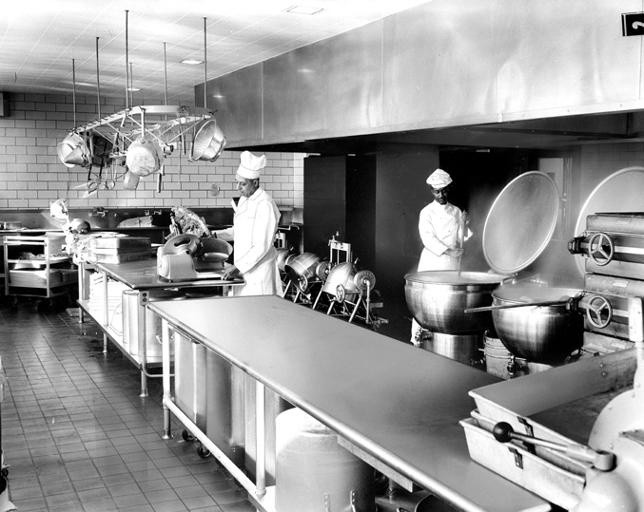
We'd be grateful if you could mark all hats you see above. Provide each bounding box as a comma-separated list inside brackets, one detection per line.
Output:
[425, 168, 454, 191]
[236, 150, 267, 179]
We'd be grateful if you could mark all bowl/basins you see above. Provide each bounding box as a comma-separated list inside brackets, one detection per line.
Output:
[200, 238, 233, 261]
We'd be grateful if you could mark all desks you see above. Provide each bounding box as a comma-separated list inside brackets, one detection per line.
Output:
[140, 293, 554, 511]
[76, 257, 249, 398]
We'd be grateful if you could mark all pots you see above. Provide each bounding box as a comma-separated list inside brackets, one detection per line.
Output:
[126, 108, 164, 177]
[490, 276, 586, 360]
[56, 131, 92, 168]
[403, 269, 512, 332]
[188, 113, 227, 163]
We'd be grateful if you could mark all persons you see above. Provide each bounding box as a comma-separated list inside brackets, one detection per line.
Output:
[408, 180, 474, 348]
[204, 170, 284, 299]
